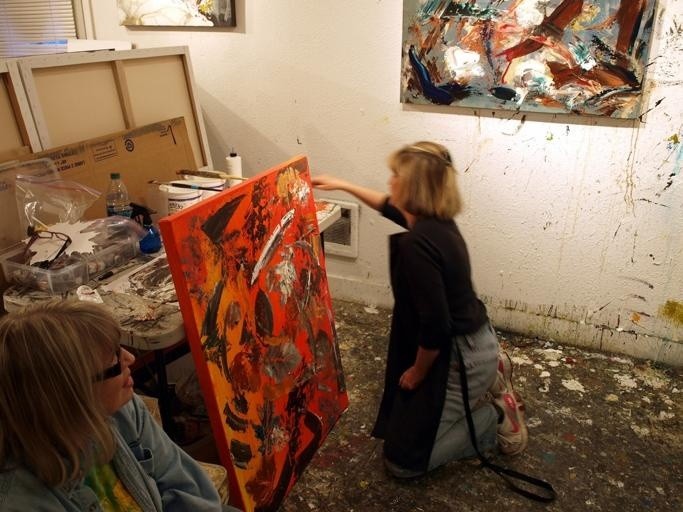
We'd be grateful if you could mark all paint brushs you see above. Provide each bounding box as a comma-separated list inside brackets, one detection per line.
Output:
[175, 169, 249, 180]
[99, 261, 136, 280]
[147, 179, 223, 192]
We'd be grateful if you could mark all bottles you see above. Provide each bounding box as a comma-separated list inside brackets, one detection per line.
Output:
[225, 147, 243, 187]
[105, 173, 132, 218]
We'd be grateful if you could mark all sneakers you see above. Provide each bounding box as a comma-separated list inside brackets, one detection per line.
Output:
[490, 350, 528, 455]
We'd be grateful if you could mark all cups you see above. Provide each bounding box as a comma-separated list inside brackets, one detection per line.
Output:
[142, 186, 169, 226]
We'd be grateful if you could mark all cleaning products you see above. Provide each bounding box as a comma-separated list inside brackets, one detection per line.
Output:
[127, 201, 162, 253]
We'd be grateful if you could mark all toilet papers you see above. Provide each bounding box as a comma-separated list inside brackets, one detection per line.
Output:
[226, 157, 242, 188]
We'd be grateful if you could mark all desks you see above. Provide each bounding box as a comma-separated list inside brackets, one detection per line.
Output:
[3, 203, 344, 444]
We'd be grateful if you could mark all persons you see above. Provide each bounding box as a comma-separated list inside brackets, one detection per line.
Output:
[0, 297, 243, 511]
[310, 140, 528, 480]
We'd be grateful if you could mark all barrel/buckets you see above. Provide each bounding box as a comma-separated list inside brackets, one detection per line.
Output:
[182, 169, 228, 198]
[156, 180, 203, 217]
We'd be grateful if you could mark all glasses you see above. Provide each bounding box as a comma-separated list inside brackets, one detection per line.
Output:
[97, 350, 122, 380]
[24, 231, 72, 265]
